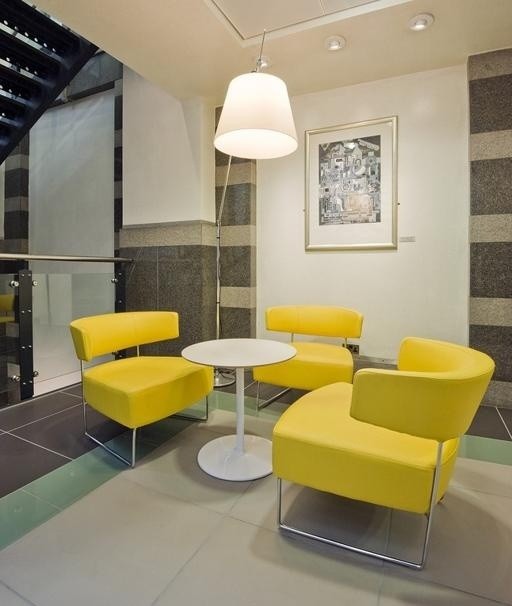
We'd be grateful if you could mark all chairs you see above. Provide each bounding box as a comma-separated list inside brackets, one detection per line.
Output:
[273, 333, 498, 573]
[70, 309, 213, 468]
[252, 304, 364, 415]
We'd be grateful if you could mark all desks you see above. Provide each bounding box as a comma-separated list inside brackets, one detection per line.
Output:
[178, 335, 297, 484]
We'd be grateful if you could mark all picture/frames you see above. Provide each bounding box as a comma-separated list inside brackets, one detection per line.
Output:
[302, 114, 399, 252]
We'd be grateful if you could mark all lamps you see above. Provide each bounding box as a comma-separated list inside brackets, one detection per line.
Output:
[409, 11, 434, 32]
[212, 28, 300, 389]
[322, 36, 347, 53]
[251, 53, 277, 69]
